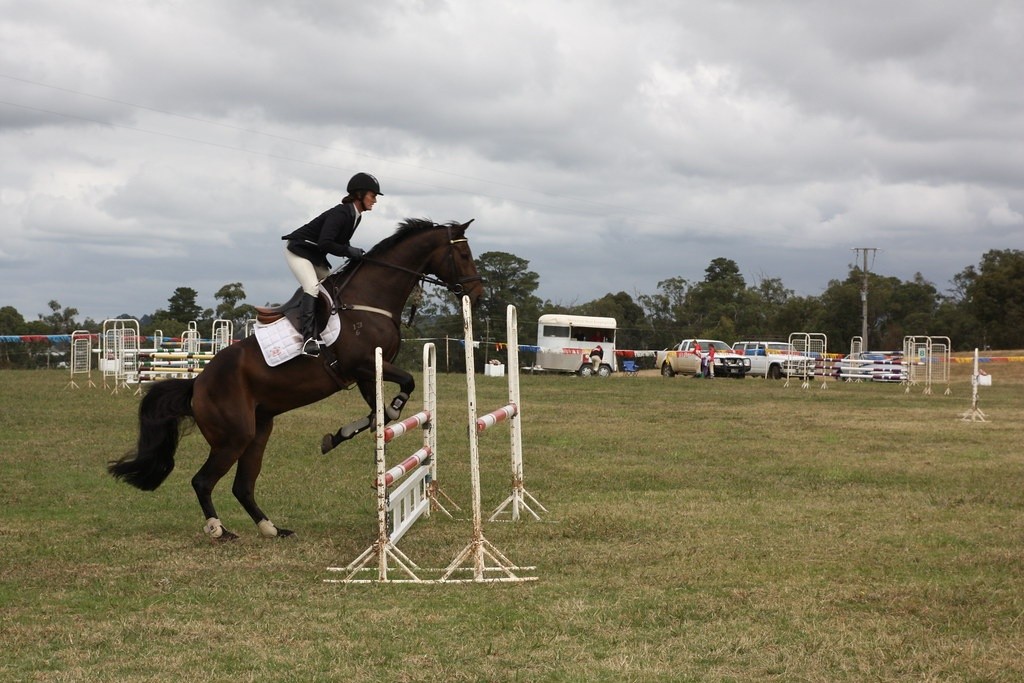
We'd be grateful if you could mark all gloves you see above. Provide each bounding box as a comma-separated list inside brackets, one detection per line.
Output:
[348, 247, 364, 258]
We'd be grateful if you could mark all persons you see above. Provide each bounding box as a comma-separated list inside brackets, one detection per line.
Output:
[282, 173, 384, 353]
[592, 331, 603, 342]
[693, 340, 701, 354]
[590, 346, 603, 375]
[708, 344, 715, 379]
[575, 329, 584, 341]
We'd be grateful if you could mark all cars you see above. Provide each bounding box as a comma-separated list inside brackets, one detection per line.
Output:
[836, 352, 908, 382]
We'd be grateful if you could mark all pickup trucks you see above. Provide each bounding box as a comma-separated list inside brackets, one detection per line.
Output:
[654, 338, 752, 380]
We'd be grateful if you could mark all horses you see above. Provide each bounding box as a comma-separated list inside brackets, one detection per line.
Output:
[108, 218, 487, 539]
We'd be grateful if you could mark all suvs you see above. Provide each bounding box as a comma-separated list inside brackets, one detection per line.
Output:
[730, 341, 814, 382]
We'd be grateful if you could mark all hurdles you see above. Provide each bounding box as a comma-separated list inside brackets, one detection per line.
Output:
[784, 331, 953, 395]
[324, 292, 548, 587]
[66, 317, 234, 397]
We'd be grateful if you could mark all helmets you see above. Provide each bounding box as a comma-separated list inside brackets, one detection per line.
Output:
[347, 173, 383, 195]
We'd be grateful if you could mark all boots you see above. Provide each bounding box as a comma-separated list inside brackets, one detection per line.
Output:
[301, 293, 321, 354]
